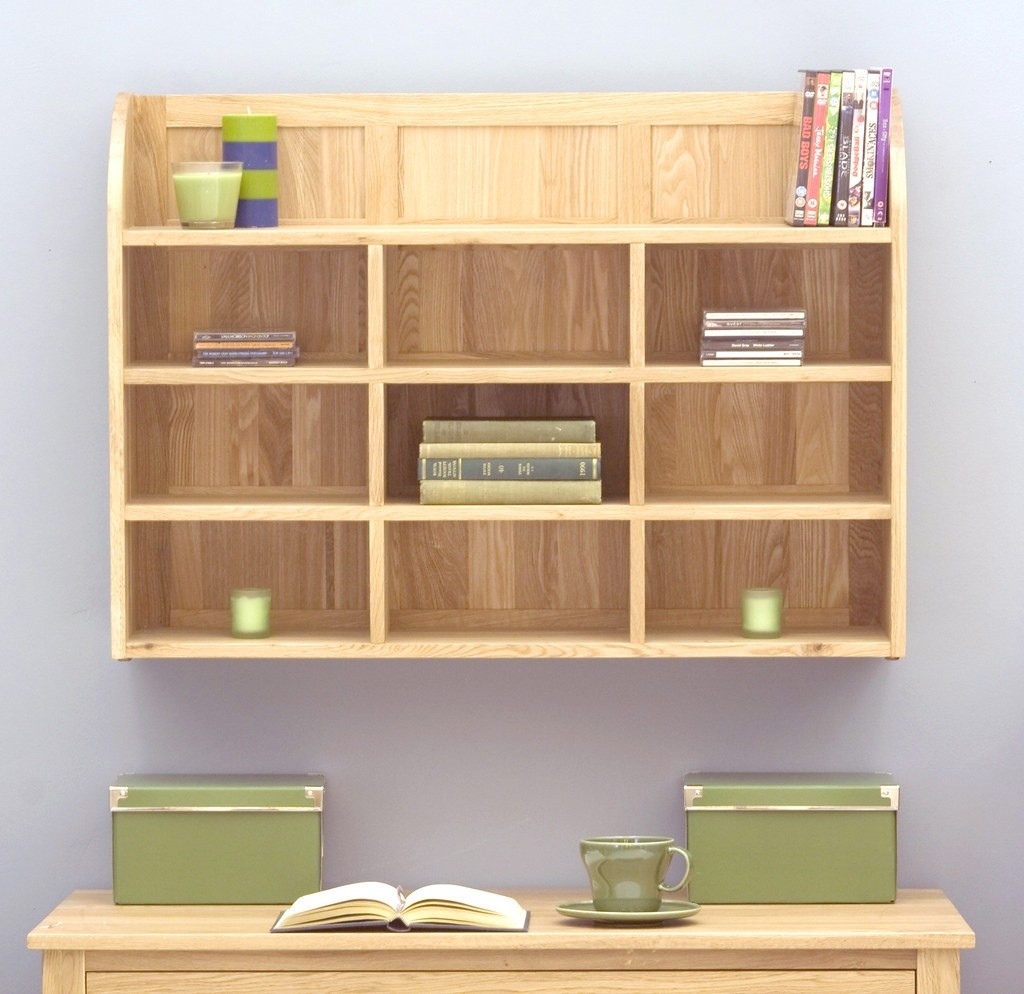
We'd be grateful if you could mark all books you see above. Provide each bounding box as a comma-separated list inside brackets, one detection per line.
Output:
[272, 882, 529, 933]
[418, 417, 603, 505]
[192, 331, 299, 366]
[786, 68, 890, 227]
[701, 309, 805, 365]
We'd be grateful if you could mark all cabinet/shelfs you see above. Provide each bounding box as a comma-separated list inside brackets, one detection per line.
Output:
[26, 888, 975, 994]
[108, 90, 907, 661]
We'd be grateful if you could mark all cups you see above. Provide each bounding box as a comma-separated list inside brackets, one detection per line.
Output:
[580, 835, 690, 912]
[740, 586, 785, 640]
[169, 159, 243, 229]
[229, 587, 270, 640]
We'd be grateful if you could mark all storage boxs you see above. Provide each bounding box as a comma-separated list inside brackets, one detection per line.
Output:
[110, 773, 325, 905]
[682, 771, 899, 904]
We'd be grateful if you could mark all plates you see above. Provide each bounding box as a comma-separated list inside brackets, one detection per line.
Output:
[555, 899, 702, 924]
